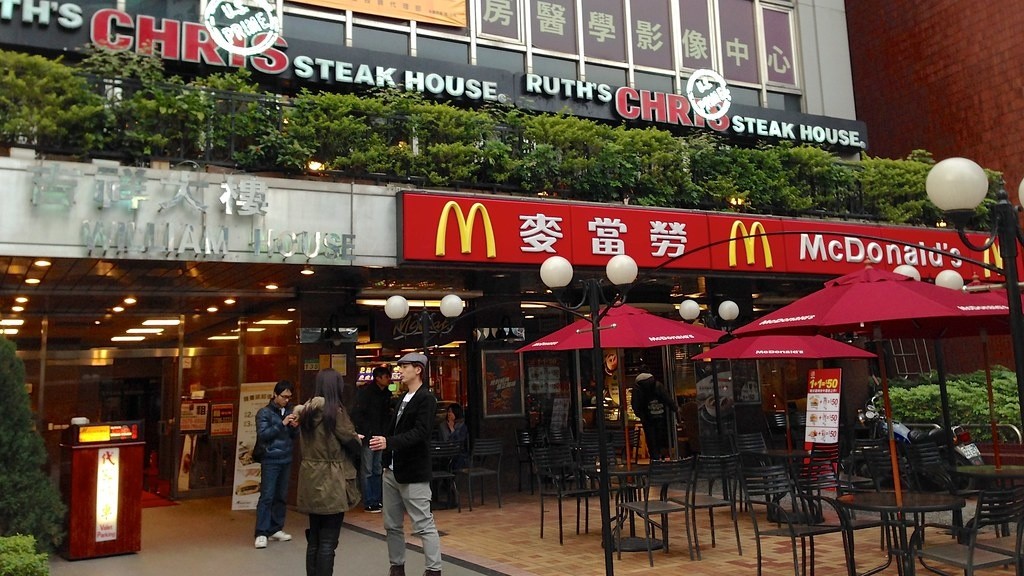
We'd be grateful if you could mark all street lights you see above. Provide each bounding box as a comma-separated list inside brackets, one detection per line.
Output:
[679, 299, 740, 500]
[539, 255, 639, 576]
[925, 157, 1024, 441]
[384, 294, 464, 390]
[893, 263, 964, 538]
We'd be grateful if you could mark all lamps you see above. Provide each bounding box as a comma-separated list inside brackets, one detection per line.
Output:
[496, 315, 524, 343]
[318, 319, 334, 341]
[484, 327, 507, 340]
[322, 312, 353, 346]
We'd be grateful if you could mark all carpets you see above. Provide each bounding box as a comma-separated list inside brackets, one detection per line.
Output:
[142, 490, 180, 507]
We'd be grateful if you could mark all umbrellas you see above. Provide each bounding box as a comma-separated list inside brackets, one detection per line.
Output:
[691, 326, 879, 512]
[733, 255, 1024, 576]
[514, 294, 728, 537]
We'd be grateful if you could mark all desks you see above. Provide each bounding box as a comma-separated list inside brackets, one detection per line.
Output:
[945, 464, 1024, 536]
[580, 464, 664, 552]
[836, 491, 966, 576]
[751, 449, 829, 525]
[548, 440, 599, 498]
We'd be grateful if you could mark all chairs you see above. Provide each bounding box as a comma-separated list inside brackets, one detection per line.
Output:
[427, 409, 1024, 576]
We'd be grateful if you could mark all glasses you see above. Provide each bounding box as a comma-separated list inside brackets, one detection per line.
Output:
[276, 392, 293, 399]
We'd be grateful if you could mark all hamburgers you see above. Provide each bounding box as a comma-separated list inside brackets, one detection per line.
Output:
[810, 414, 817, 422]
[235, 480, 261, 495]
[810, 398, 819, 407]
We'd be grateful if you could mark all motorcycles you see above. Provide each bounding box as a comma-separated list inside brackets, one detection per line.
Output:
[849, 390, 984, 500]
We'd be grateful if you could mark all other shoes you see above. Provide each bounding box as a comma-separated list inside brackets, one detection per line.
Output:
[363, 506, 382, 513]
[389, 563, 409, 576]
[424, 570, 443, 576]
[268, 530, 295, 542]
[254, 535, 269, 548]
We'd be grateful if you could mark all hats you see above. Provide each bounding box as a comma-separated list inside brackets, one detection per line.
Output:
[396, 353, 433, 367]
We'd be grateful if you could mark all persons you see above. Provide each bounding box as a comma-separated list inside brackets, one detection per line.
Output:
[293, 368, 362, 576]
[586, 379, 596, 396]
[350, 367, 391, 513]
[632, 365, 681, 453]
[433, 403, 467, 507]
[357, 353, 441, 576]
[254, 380, 299, 548]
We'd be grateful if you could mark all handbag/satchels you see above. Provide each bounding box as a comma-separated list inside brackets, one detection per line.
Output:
[251, 433, 264, 463]
